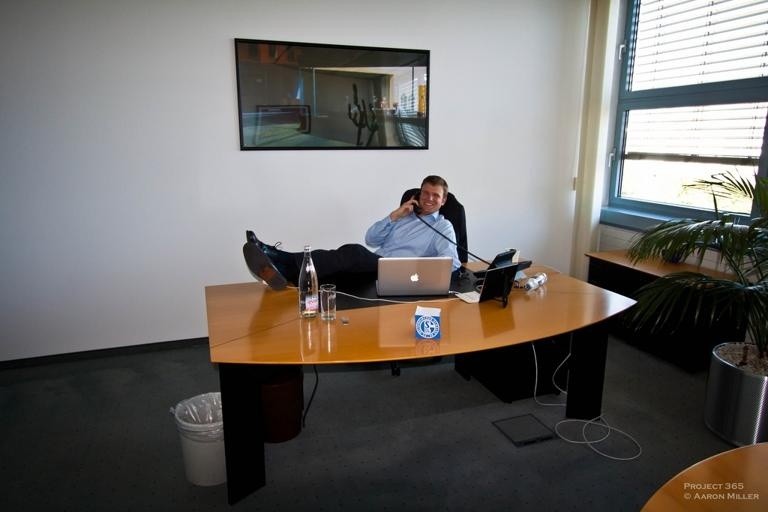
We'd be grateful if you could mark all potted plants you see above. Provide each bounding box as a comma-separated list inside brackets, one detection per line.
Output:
[628, 171, 768, 446]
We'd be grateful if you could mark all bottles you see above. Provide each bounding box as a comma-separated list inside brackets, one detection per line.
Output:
[299, 246, 319, 318]
[523, 272, 548, 289]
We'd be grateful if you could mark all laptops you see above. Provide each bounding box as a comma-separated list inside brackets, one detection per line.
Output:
[377, 301, 451, 349]
[374, 255, 454, 298]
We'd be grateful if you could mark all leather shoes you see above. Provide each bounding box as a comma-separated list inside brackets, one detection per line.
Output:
[243, 230, 287, 291]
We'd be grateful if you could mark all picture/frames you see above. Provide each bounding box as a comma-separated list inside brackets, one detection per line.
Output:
[253, 105, 312, 147]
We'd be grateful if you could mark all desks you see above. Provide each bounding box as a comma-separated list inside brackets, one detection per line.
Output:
[205, 256, 639, 505]
[584, 246, 750, 376]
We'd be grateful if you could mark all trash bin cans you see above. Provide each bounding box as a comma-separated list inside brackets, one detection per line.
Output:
[169, 392, 228, 487]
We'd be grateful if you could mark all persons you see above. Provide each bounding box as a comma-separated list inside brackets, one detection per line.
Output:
[243, 172, 464, 290]
[392, 101, 408, 118]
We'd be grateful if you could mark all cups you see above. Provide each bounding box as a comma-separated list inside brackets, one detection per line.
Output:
[318, 283, 336, 321]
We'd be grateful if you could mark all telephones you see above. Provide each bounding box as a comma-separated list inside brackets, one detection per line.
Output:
[414, 191, 422, 216]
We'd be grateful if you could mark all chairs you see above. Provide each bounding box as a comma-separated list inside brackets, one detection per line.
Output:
[400, 187, 468, 264]
